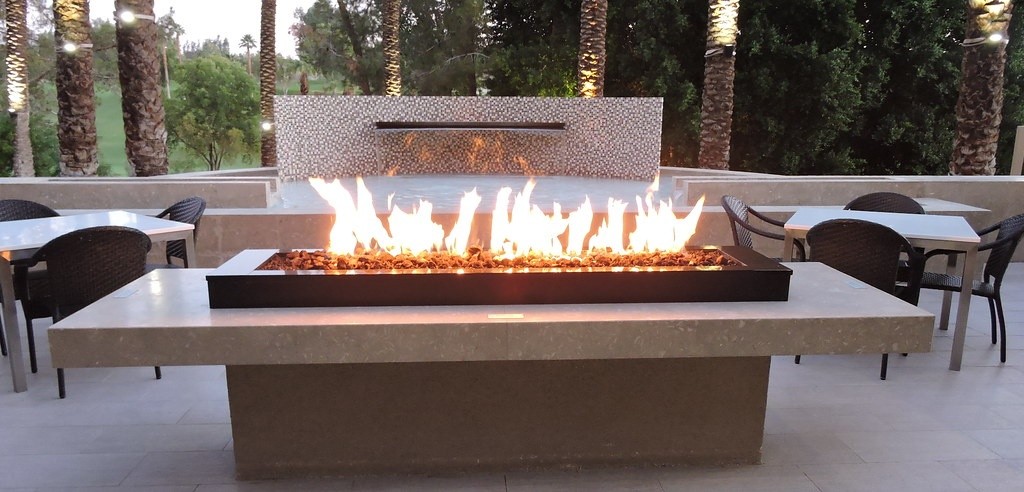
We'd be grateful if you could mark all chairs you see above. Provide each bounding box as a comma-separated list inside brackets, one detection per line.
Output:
[145, 197, 207, 273]
[720, 195, 807, 263]
[794, 219, 928, 381]
[14, 225, 162, 400]
[0, 198, 62, 358]
[843, 191, 924, 280]
[922, 214, 1024, 362]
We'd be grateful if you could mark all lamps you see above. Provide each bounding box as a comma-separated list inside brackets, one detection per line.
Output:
[984, 0, 1005, 12]
[724, 43, 735, 58]
[989, 31, 1003, 41]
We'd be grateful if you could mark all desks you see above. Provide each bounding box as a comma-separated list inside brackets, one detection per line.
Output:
[781, 205, 983, 373]
[0, 211, 195, 393]
